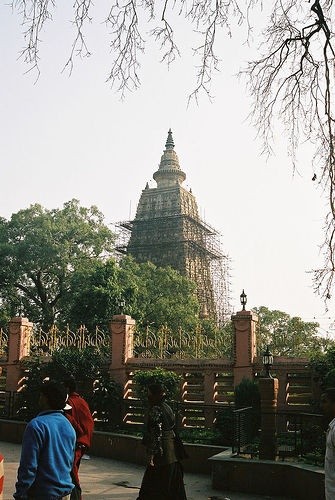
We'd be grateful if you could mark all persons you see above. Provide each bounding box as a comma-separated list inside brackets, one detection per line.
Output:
[12, 381, 78, 500]
[134, 382, 187, 500]
[53, 373, 95, 500]
[323, 418, 335, 499]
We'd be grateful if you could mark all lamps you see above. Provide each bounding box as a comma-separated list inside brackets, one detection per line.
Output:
[239, 288, 248, 311]
[263, 344, 273, 378]
